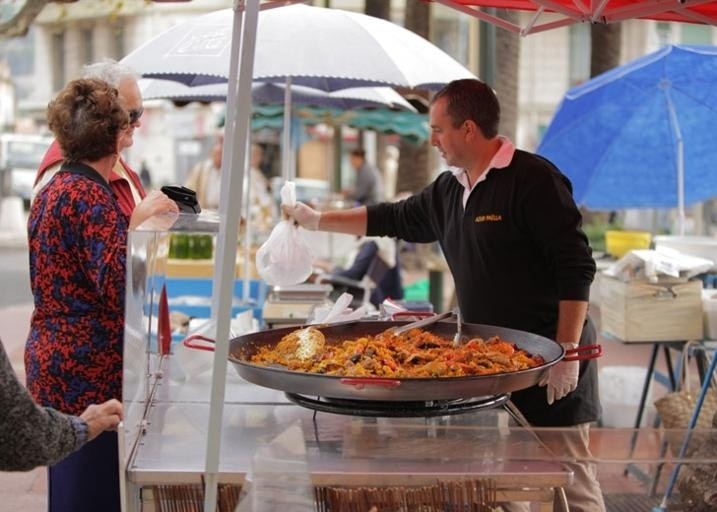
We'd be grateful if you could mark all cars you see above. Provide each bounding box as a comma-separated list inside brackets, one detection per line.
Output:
[4, 128, 151, 213]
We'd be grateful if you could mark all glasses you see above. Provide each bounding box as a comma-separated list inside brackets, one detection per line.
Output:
[130, 108, 143, 119]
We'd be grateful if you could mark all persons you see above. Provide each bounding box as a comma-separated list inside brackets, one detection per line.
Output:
[280, 80, 607, 512]
[0, 338, 124, 472]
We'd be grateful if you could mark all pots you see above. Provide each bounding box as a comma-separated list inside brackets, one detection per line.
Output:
[182, 306, 605, 405]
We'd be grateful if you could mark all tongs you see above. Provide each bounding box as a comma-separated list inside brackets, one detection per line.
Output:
[395, 309, 463, 348]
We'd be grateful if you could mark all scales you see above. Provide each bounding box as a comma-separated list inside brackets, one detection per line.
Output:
[262, 283, 334, 330]
